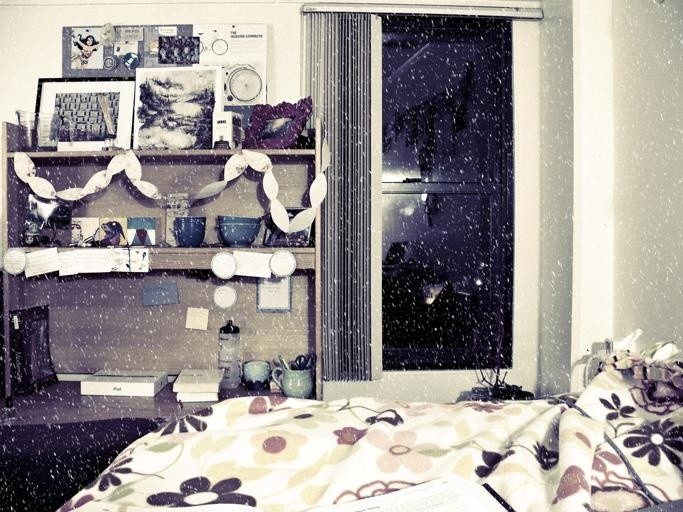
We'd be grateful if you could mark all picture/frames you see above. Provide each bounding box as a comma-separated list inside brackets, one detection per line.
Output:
[33, 77, 136, 150]
[133, 65, 225, 150]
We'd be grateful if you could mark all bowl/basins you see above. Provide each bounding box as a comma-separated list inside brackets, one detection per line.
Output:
[217, 223, 261, 243]
[217, 215, 260, 227]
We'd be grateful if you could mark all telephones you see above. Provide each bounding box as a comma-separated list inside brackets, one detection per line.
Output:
[262, 207, 313, 246]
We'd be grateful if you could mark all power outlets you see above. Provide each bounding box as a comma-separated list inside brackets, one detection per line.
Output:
[591, 342, 607, 358]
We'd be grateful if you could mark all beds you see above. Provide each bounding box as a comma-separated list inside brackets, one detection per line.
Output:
[53, 353, 683, 512]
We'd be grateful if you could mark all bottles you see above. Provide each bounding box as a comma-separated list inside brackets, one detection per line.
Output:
[218, 318, 240, 388]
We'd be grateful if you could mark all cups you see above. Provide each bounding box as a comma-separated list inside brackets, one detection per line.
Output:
[176, 216, 207, 249]
[244, 361, 270, 385]
[272, 363, 313, 399]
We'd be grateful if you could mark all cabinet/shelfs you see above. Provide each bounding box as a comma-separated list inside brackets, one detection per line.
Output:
[0, 118, 321, 511]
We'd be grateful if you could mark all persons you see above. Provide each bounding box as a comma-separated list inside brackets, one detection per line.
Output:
[159, 37, 199, 64]
[71, 35, 103, 69]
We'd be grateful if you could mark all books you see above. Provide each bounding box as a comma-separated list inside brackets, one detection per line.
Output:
[171, 368, 225, 403]
[10, 303, 59, 395]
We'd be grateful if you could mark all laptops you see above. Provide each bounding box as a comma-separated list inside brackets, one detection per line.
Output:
[382, 241, 408, 268]
